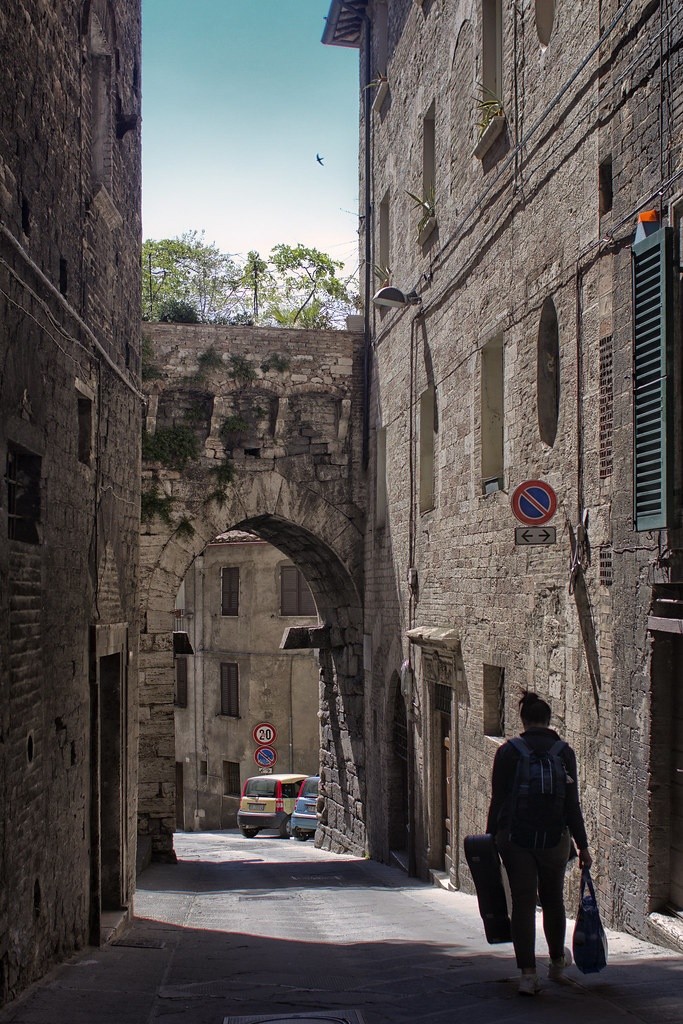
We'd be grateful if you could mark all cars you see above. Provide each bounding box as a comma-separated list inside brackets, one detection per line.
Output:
[290, 776, 320, 841]
[237, 773, 310, 840]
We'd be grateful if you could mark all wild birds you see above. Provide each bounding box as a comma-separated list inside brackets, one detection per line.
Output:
[316, 153, 324, 166]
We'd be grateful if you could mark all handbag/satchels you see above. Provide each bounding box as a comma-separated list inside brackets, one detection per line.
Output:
[573, 865, 607, 974]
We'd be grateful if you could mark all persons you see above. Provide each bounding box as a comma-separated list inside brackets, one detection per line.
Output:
[486, 689, 592, 997]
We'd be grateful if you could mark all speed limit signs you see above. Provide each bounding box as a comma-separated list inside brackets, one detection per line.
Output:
[253, 723, 277, 745]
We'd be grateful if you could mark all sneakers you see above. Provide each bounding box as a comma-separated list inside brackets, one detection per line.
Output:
[519, 973, 545, 993]
[547, 947, 572, 980]
